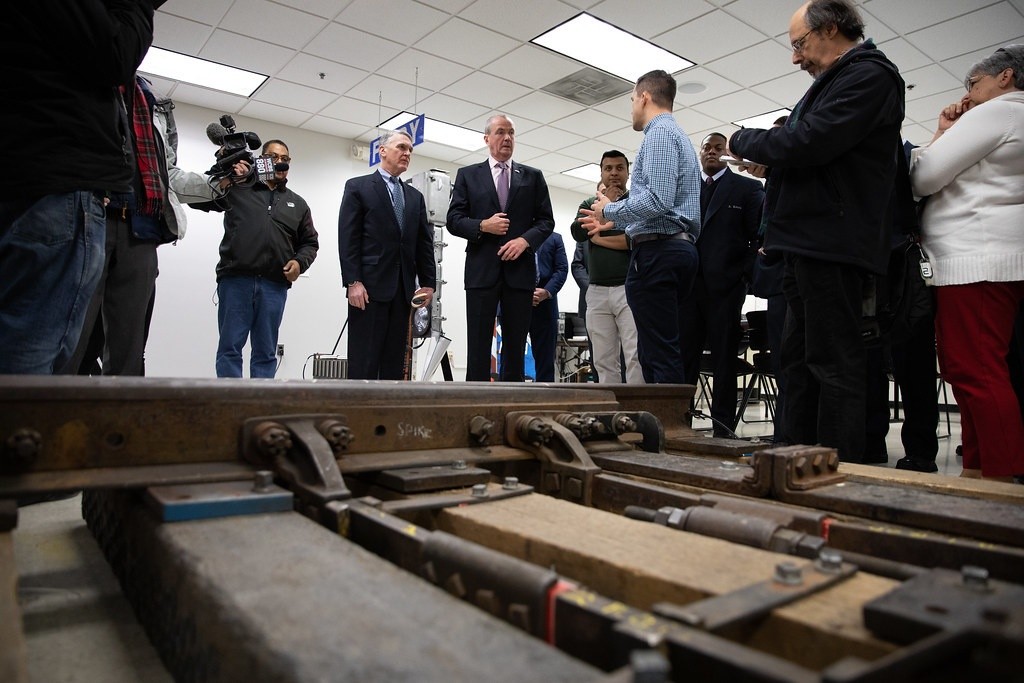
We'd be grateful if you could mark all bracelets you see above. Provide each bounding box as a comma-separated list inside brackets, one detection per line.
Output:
[480, 221, 482, 231]
[602, 208, 605, 219]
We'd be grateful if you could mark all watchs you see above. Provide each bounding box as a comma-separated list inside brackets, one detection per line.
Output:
[349, 281, 358, 287]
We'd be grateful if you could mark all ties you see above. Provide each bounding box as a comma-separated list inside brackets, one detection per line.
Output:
[707, 177, 713, 185]
[389, 176, 404, 233]
[495, 162, 510, 213]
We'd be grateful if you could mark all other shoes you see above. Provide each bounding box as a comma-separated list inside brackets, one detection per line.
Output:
[897, 455, 940, 473]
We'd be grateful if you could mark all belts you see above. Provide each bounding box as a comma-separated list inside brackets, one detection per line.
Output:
[106, 203, 129, 221]
[630, 232, 691, 250]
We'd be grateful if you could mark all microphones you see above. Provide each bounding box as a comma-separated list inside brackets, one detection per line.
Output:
[253, 156, 290, 181]
[206, 123, 228, 146]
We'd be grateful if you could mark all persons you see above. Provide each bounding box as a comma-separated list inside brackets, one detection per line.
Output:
[699, 132, 765, 439]
[590, 69, 700, 383]
[0, 0, 186, 378]
[497, 232, 568, 383]
[887, 131, 939, 473]
[338, 129, 436, 381]
[446, 115, 555, 383]
[571, 181, 626, 383]
[909, 44, 1024, 483]
[570, 148, 645, 383]
[727, 0, 906, 463]
[187, 140, 319, 378]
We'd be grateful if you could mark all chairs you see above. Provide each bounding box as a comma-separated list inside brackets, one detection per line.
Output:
[741, 309, 785, 424]
[694, 319, 751, 418]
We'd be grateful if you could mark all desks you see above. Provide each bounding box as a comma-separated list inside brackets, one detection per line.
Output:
[557, 341, 589, 383]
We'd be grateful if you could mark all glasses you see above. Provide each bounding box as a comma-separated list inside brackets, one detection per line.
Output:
[262, 152, 291, 164]
[967, 72, 992, 91]
[792, 25, 823, 52]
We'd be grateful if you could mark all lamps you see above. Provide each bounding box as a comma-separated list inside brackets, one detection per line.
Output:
[350, 144, 369, 161]
